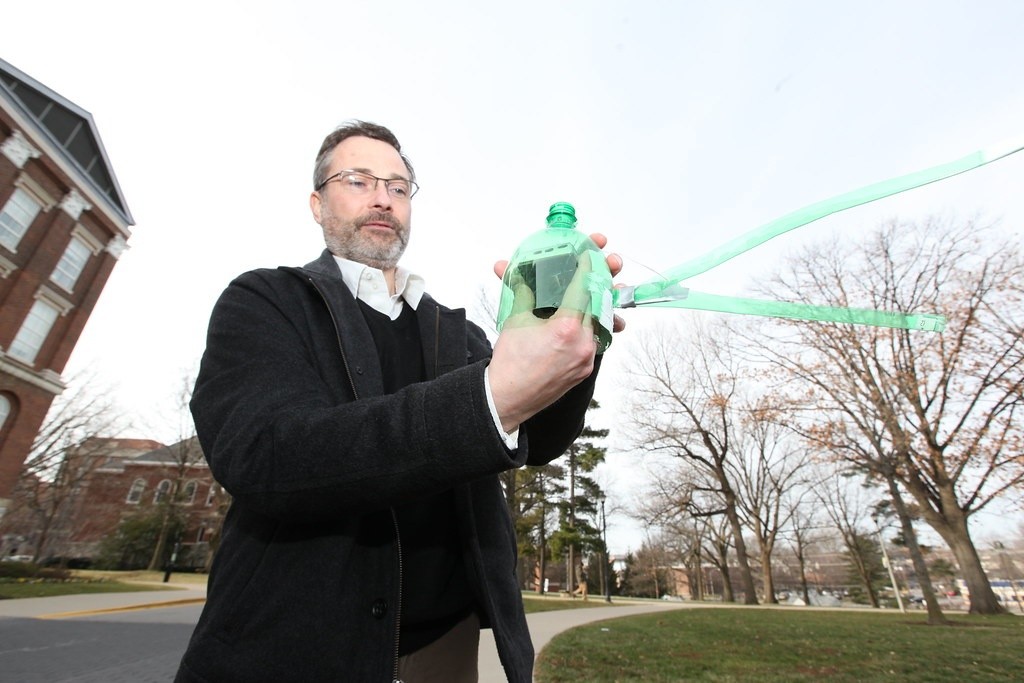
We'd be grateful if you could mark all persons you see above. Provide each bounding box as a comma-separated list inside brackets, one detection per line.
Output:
[572, 562, 588, 601]
[172, 120, 629, 683]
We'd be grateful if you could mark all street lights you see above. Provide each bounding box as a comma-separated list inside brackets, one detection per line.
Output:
[870, 512, 904, 612]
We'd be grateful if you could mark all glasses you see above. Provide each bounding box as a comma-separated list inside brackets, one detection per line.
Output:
[315, 169, 419, 200]
